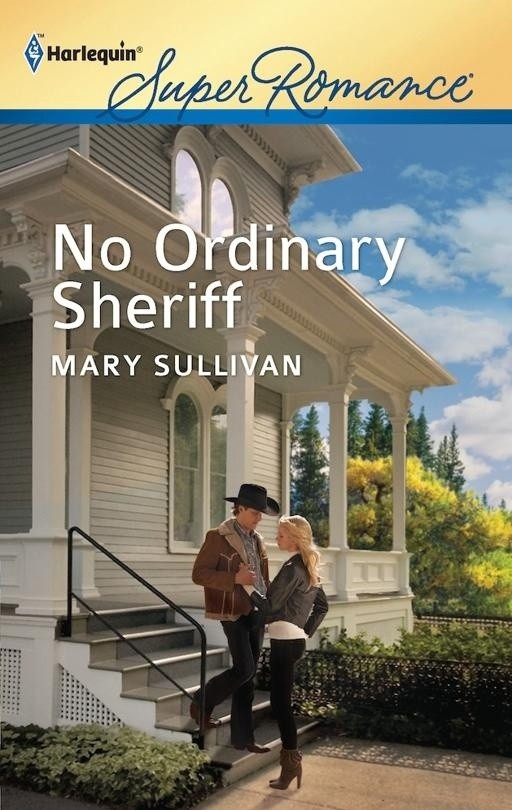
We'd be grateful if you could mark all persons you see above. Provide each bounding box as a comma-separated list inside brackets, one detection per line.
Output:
[181, 483, 280, 753]
[239, 513, 328, 792]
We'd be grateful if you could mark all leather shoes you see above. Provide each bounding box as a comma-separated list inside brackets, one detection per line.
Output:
[234, 742, 271, 753]
[190, 703, 222, 728]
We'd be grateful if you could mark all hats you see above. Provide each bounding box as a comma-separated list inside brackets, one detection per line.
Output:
[224, 484, 280, 516]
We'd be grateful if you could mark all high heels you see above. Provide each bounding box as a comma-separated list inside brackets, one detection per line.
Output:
[269, 749, 302, 789]
